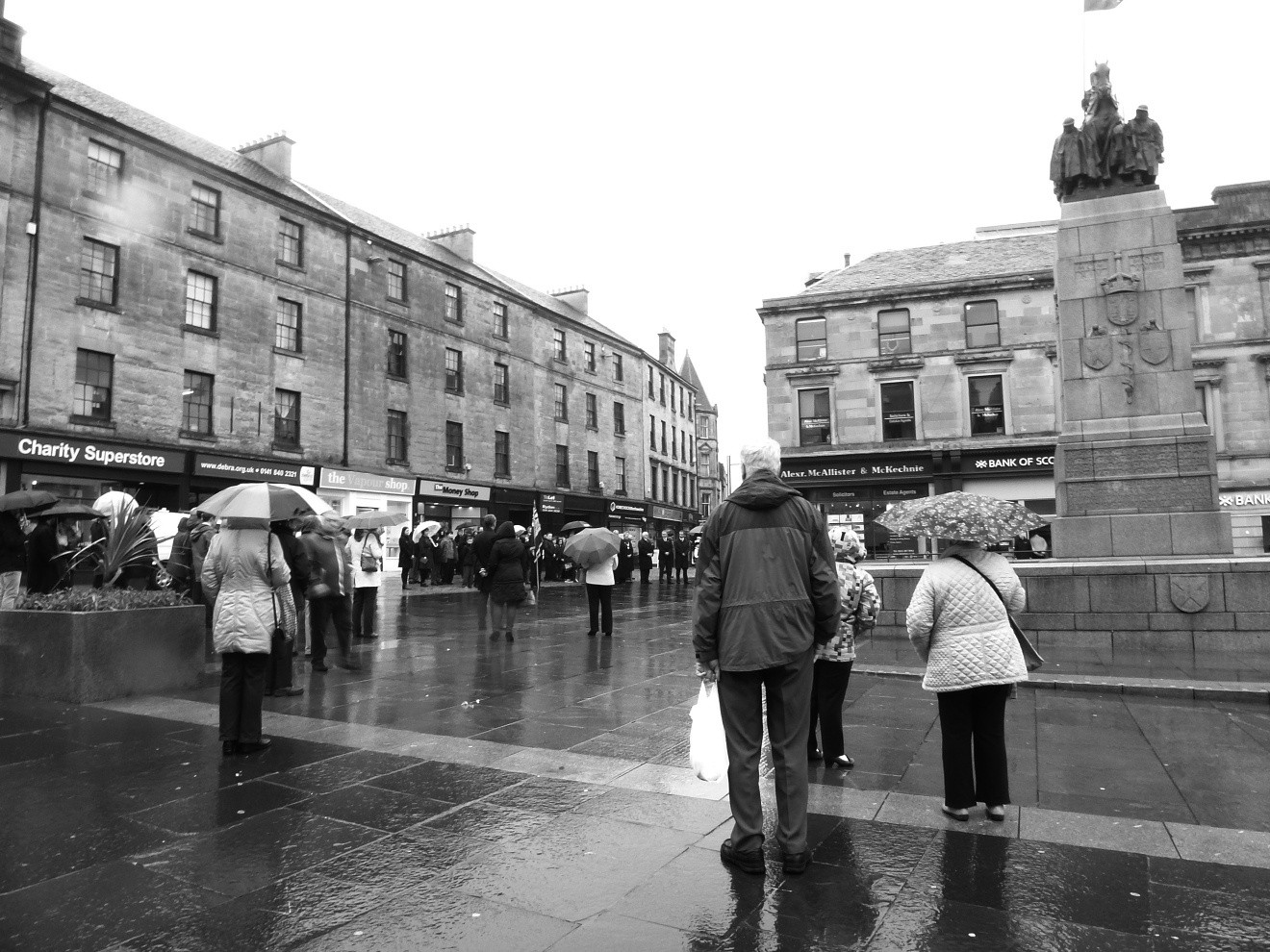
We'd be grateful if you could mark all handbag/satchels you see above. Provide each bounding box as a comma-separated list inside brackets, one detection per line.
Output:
[360, 532, 379, 571]
[517, 589, 535, 609]
[1008, 613, 1044, 672]
[272, 625, 286, 660]
[305, 577, 339, 600]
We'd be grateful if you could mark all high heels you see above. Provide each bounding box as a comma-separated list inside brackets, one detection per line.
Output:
[808, 750, 823, 762]
[587, 629, 596, 637]
[823, 753, 855, 768]
[604, 631, 611, 637]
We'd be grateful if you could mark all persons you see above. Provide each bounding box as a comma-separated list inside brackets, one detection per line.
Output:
[1030, 529, 1048, 558]
[0, 506, 704, 697]
[691, 436, 841, 875]
[1014, 534, 1031, 558]
[199, 517, 292, 756]
[1049, 73, 1165, 195]
[807, 526, 881, 767]
[906, 541, 1030, 821]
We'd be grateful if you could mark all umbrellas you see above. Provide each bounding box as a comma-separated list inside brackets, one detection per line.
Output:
[0, 490, 60, 524]
[194, 481, 334, 520]
[27, 503, 105, 531]
[91, 486, 140, 517]
[872, 489, 1051, 544]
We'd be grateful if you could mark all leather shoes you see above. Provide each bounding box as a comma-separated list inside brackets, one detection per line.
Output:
[720, 838, 766, 873]
[234, 737, 272, 754]
[222, 740, 236, 755]
[782, 849, 810, 873]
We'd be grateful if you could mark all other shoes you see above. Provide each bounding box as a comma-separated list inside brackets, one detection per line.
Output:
[478, 625, 486, 631]
[262, 687, 273, 695]
[985, 804, 1004, 821]
[941, 803, 969, 821]
[292, 629, 380, 656]
[398, 571, 703, 591]
[312, 660, 328, 671]
[339, 655, 359, 668]
[489, 630, 500, 641]
[506, 632, 514, 642]
[274, 686, 303, 697]
